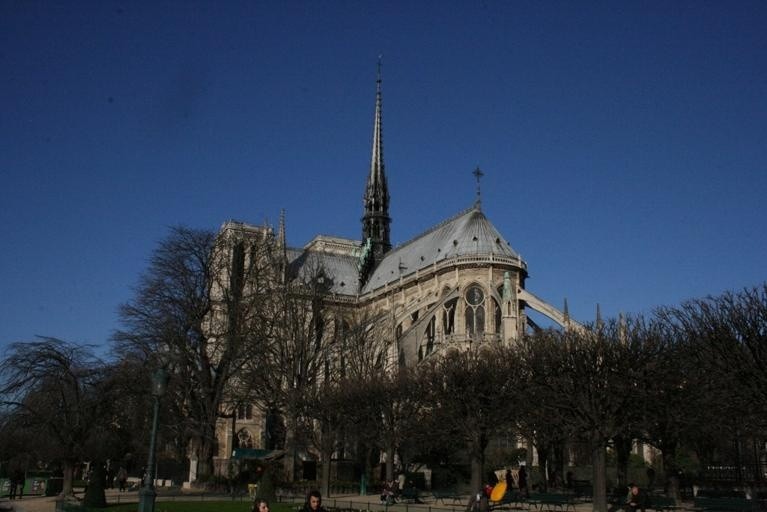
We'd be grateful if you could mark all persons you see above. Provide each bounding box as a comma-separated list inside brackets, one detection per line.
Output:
[17, 472, 25, 499]
[518, 466, 531, 498]
[608, 463, 690, 512]
[506, 470, 515, 497]
[475, 489, 490, 512]
[84, 456, 115, 492]
[299, 490, 326, 512]
[117, 466, 128, 491]
[380, 479, 416, 505]
[548, 465, 561, 488]
[252, 498, 271, 512]
[488, 466, 498, 487]
[138, 466, 147, 488]
[8, 470, 19, 499]
[29, 460, 63, 493]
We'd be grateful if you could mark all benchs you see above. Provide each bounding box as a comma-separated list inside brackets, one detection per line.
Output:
[431, 491, 467, 506]
[486, 491, 584, 512]
[689, 496, 761, 512]
[400, 496, 428, 504]
[607, 488, 676, 512]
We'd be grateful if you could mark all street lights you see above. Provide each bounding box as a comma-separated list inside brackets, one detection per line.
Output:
[135, 366, 173, 512]
